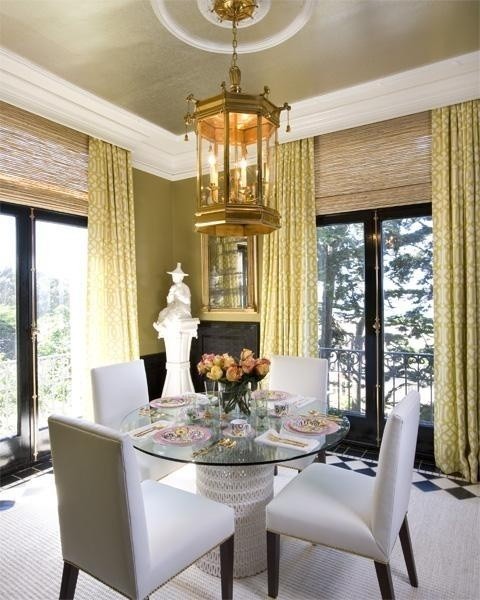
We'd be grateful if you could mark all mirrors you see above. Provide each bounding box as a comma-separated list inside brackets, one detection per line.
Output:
[198, 233, 258, 311]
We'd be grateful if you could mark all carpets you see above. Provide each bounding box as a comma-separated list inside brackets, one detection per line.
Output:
[0, 473, 479, 599]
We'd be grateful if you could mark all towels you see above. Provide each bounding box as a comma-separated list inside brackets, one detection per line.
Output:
[127, 420, 171, 442]
[254, 428, 319, 453]
[289, 395, 316, 409]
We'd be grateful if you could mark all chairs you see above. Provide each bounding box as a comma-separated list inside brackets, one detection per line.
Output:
[268, 355, 329, 477]
[90, 358, 175, 483]
[47, 414, 235, 600]
[265, 390, 421, 600]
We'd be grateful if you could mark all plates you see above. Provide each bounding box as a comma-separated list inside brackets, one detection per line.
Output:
[131, 389, 340, 457]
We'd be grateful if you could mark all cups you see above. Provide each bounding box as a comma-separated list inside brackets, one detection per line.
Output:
[217, 381, 225, 393]
[204, 381, 216, 397]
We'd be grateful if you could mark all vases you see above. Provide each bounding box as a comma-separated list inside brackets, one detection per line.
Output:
[216, 381, 250, 420]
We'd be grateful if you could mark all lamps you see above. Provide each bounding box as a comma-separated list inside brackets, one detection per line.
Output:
[179, 0, 291, 239]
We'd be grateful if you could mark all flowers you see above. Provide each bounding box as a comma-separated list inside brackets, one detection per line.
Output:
[196, 348, 271, 417]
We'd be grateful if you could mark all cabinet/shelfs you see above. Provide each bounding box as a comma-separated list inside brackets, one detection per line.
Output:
[190, 323, 260, 395]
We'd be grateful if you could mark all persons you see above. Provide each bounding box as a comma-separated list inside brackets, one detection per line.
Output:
[153, 262, 193, 333]
